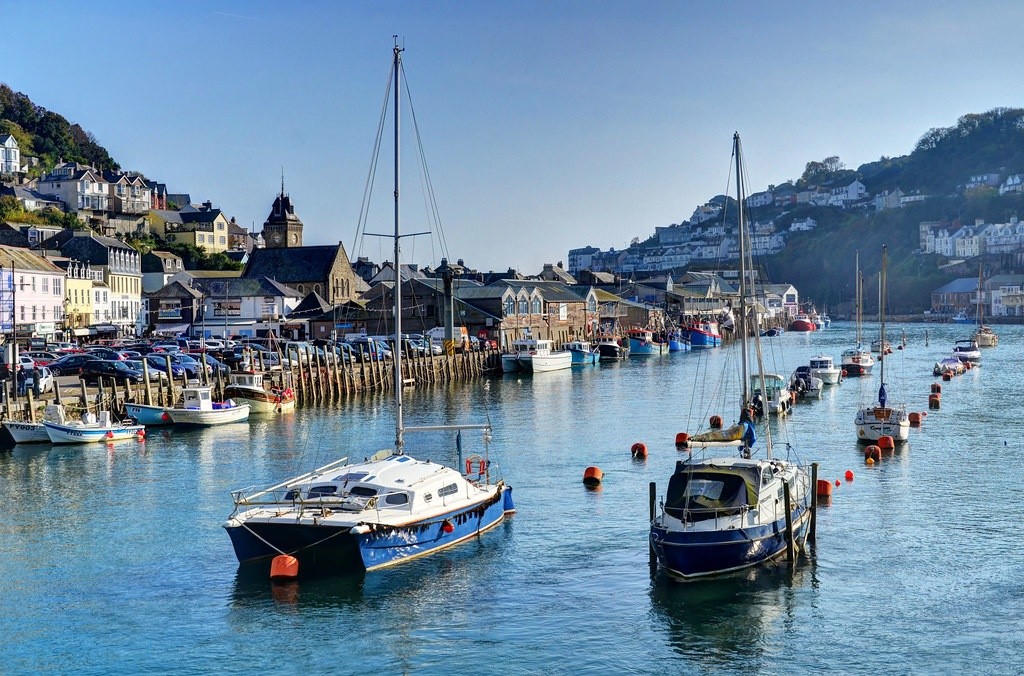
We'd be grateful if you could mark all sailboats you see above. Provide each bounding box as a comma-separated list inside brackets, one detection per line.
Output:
[221, 31, 518, 584]
[853, 243, 911, 448]
[835, 255, 876, 376]
[870, 269, 891, 353]
[969, 261, 999, 348]
[647, 130, 818, 585]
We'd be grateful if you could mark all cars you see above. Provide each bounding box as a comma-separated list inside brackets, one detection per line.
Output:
[79, 360, 143, 387]
[183, 352, 232, 376]
[125, 355, 186, 380]
[147, 352, 214, 379]
[45, 353, 102, 377]
[17, 366, 54, 396]
[0, 326, 499, 372]
[114, 360, 169, 384]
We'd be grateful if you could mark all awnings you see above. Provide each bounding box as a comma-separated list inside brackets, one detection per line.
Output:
[153, 323, 190, 333]
[73, 326, 122, 336]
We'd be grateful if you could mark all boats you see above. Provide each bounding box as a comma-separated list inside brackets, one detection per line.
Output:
[933, 351, 965, 377]
[502, 326, 573, 374]
[738, 373, 797, 420]
[592, 312, 631, 363]
[2, 418, 124, 449]
[953, 309, 981, 325]
[124, 358, 252, 429]
[618, 294, 725, 356]
[786, 365, 824, 399]
[41, 408, 145, 446]
[223, 367, 295, 415]
[952, 336, 981, 362]
[758, 296, 832, 338]
[809, 350, 843, 385]
[561, 330, 601, 365]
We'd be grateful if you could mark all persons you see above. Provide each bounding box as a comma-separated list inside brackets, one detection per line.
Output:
[72, 336, 91, 348]
[17, 365, 28, 397]
[33, 367, 40, 399]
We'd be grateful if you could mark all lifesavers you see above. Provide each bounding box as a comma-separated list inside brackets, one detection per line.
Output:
[466, 454, 485, 475]
[282, 388, 292, 399]
